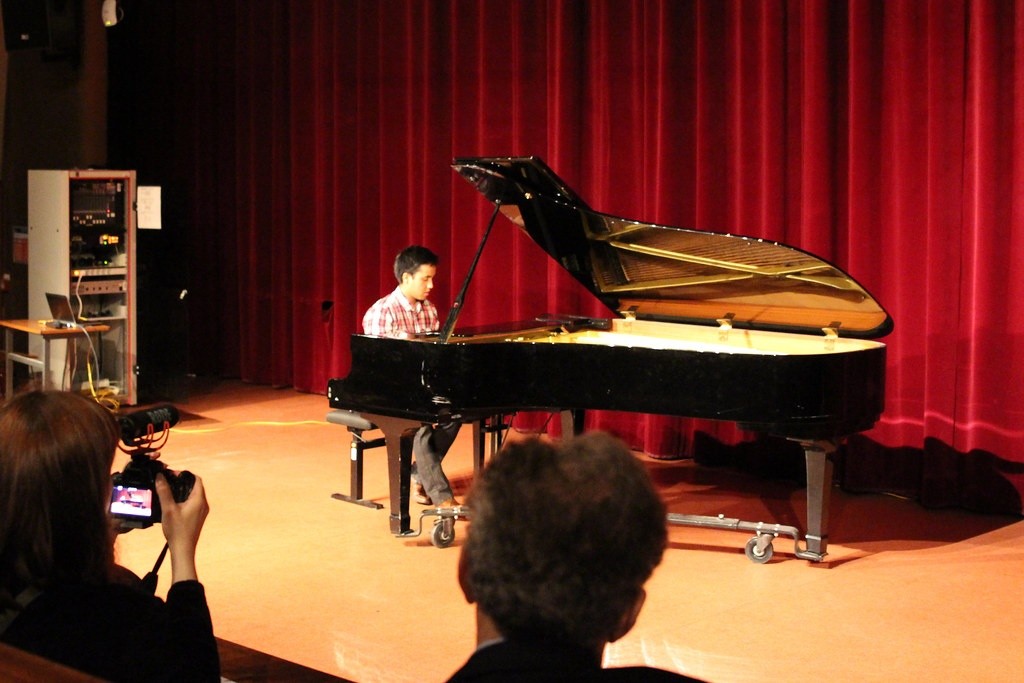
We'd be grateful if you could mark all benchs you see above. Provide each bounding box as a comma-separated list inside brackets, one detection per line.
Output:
[326, 410, 388, 510]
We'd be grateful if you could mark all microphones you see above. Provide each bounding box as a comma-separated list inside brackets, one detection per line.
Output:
[119, 404, 181, 439]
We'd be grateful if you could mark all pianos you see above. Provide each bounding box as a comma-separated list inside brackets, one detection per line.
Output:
[327, 152, 896, 566]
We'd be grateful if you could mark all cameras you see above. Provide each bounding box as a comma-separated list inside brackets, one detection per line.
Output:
[107, 452, 196, 529]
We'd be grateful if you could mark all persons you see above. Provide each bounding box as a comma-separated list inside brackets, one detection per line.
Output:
[444, 433, 713, 683]
[0, 389, 221, 683]
[361, 244, 468, 521]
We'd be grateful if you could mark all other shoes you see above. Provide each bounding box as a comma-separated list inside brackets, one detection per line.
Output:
[410, 461, 431, 505]
[437, 497, 469, 522]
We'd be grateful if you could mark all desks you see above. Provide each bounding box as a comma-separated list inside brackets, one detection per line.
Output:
[0, 318, 110, 400]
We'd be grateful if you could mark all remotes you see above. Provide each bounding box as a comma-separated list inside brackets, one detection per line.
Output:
[46, 321, 62, 329]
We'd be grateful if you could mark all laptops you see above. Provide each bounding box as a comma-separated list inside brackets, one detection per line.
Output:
[45, 293, 102, 328]
